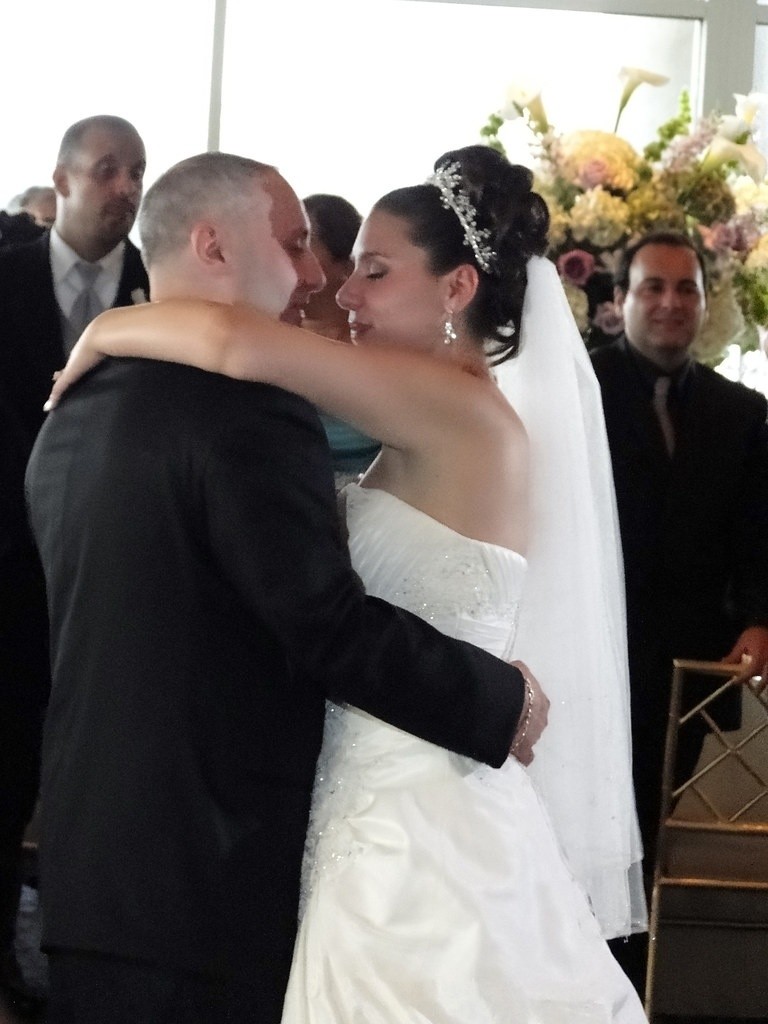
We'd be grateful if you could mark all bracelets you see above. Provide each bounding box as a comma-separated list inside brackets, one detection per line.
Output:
[510, 678, 533, 751]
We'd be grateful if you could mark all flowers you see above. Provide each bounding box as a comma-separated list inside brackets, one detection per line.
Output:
[475, 71, 768, 378]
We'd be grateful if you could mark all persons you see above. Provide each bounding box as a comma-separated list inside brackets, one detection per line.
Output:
[14, 185, 57, 228]
[301, 195, 381, 492]
[14, 151, 550, 1024]
[44, 146, 651, 1024]
[591, 230, 768, 1004]
[0, 117, 147, 1020]
[556, 249, 617, 372]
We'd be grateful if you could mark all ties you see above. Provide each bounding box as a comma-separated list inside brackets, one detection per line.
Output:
[653, 376, 677, 461]
[62, 262, 108, 335]
